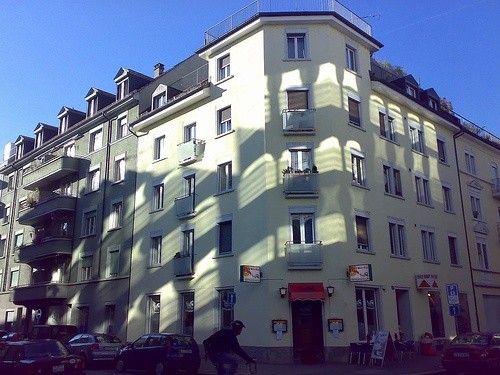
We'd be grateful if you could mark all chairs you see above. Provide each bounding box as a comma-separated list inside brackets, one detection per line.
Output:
[348, 340, 415, 365]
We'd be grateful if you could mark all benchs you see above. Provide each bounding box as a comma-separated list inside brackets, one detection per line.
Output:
[459, 338, 487, 343]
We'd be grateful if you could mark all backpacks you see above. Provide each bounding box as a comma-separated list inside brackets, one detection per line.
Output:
[203, 329, 226, 360]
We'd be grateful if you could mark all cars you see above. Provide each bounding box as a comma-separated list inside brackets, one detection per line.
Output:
[441, 332, 500, 375]
[0, 331, 84, 375]
[68, 333, 125, 368]
[115, 333, 201, 375]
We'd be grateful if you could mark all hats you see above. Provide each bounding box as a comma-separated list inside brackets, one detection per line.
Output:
[232, 320, 246, 329]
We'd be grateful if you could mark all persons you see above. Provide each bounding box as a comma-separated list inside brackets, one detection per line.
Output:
[206, 319, 257, 375]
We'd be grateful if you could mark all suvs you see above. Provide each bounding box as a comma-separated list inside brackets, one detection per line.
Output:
[27, 324, 77, 339]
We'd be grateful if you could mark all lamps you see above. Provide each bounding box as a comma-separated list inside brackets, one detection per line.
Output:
[327, 286, 335, 297]
[280, 287, 287, 298]
